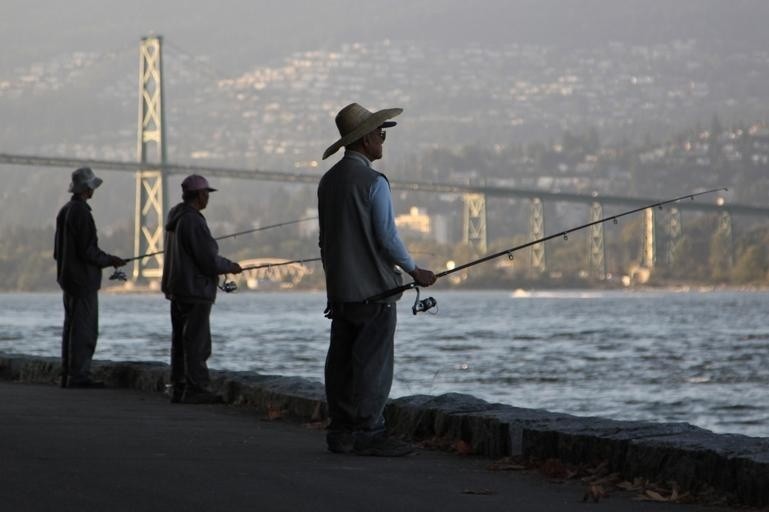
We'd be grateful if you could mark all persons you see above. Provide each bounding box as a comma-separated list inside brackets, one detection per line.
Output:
[159, 174, 243, 405]
[316, 102, 439, 455]
[52, 165, 128, 389]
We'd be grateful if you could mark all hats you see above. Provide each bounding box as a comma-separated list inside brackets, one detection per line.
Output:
[68, 167, 103, 194]
[322, 103, 403, 160]
[181, 175, 217, 193]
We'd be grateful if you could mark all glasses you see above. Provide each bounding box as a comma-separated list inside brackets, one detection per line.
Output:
[373, 130, 387, 142]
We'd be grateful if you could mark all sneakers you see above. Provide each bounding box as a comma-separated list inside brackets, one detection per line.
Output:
[170, 389, 224, 404]
[326, 429, 417, 458]
[60, 375, 104, 388]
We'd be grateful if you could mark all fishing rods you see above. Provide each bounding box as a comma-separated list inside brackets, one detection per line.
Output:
[352, 188, 727, 314]
[110, 216, 319, 281]
[217, 257, 321, 293]
